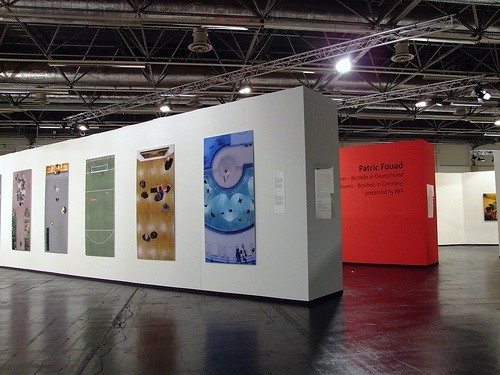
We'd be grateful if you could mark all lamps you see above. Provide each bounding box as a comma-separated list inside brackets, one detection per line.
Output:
[160, 99, 173, 112]
[239, 79, 252, 93]
[79, 121, 89, 130]
[475, 89, 491, 100]
